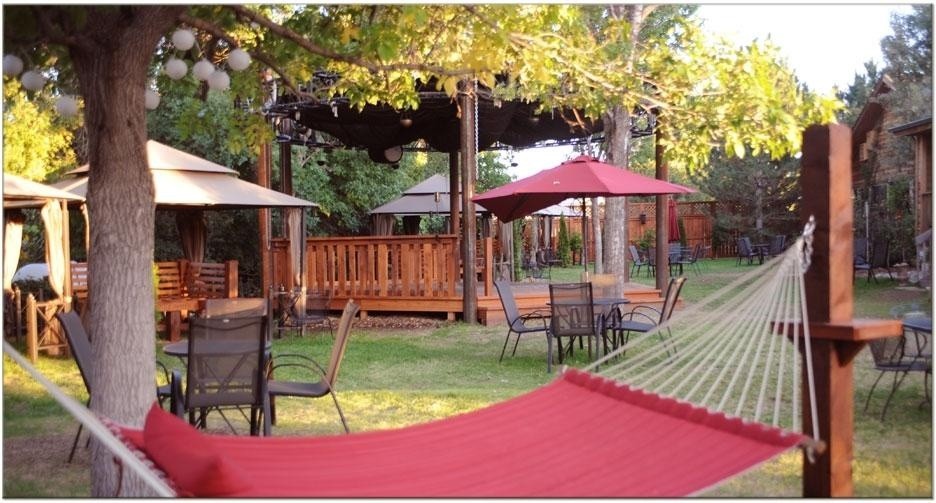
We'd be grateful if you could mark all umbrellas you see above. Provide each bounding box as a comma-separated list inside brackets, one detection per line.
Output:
[668, 194, 681, 245]
[471, 154, 697, 358]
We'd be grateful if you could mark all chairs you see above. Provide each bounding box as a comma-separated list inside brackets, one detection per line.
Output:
[490, 272, 688, 376]
[627, 232, 798, 279]
[205, 300, 275, 425]
[257, 301, 357, 438]
[69, 287, 332, 346]
[854, 228, 895, 283]
[182, 308, 264, 440]
[862, 329, 931, 420]
[52, 308, 173, 462]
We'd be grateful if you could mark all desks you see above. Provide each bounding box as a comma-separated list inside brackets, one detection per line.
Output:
[891, 316, 932, 411]
[165, 337, 274, 436]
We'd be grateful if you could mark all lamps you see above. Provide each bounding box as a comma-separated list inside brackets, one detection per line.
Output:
[398, 106, 416, 128]
